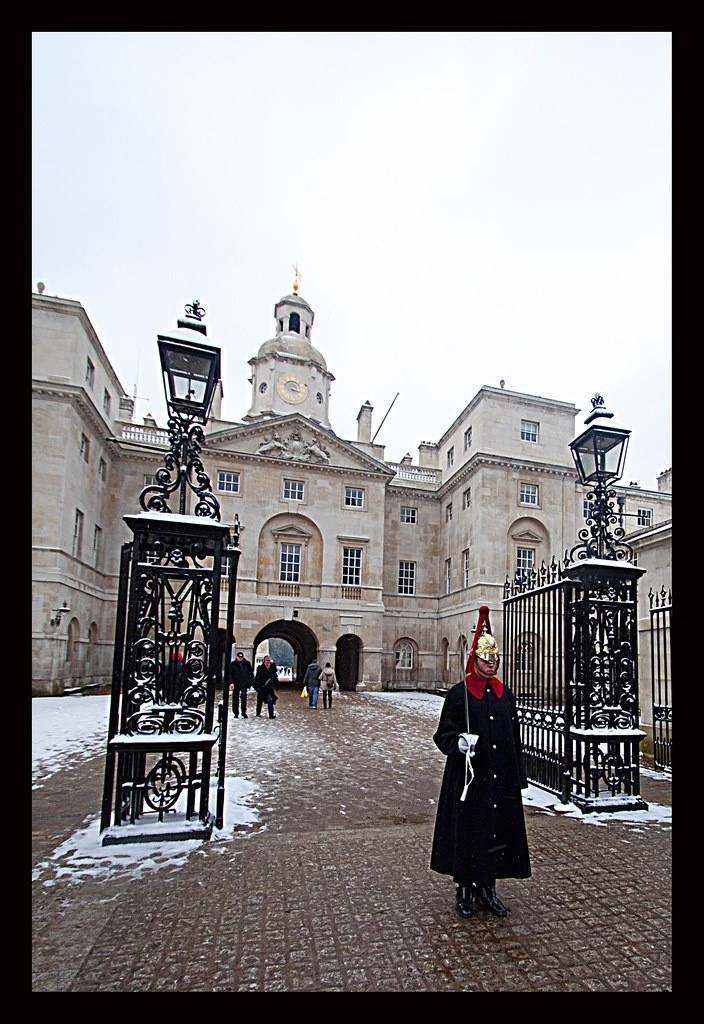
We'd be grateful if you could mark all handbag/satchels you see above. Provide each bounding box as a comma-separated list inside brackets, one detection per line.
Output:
[327, 681, 333, 688]
[301, 685, 308, 698]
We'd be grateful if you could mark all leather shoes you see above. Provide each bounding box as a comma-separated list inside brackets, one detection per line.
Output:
[456, 885, 476, 919]
[476, 884, 507, 917]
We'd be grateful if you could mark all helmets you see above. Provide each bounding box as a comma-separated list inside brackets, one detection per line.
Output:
[465, 605, 500, 678]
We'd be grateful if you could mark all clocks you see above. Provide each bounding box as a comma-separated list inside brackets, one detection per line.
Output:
[276, 372, 308, 403]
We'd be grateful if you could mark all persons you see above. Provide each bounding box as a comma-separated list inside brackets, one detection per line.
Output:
[318, 662, 337, 709]
[430, 604, 533, 919]
[157, 652, 185, 730]
[229, 652, 254, 719]
[252, 655, 280, 719]
[303, 659, 322, 709]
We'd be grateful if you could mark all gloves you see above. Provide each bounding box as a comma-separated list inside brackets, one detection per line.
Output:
[458, 737, 469, 754]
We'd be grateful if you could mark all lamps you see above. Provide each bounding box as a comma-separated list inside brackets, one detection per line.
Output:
[50, 599, 70, 626]
[568, 392, 631, 558]
[139, 299, 224, 525]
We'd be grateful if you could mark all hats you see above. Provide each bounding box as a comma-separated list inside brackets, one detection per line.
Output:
[172, 652, 184, 662]
[237, 652, 243, 657]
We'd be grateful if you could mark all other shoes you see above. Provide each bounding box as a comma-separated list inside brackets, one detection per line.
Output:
[242, 713, 248, 719]
[234, 714, 238, 719]
[256, 714, 261, 717]
[269, 715, 276, 719]
[309, 705, 317, 709]
